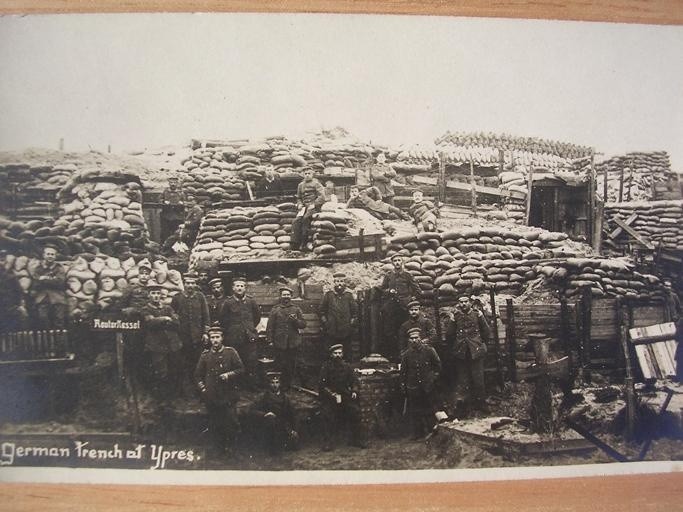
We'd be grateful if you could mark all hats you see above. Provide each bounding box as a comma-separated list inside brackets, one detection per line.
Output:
[146, 284, 164, 293]
[262, 150, 425, 198]
[390, 253, 404, 262]
[402, 293, 472, 336]
[265, 371, 283, 382]
[278, 286, 294, 294]
[138, 265, 152, 276]
[328, 342, 345, 353]
[207, 327, 226, 336]
[207, 277, 223, 287]
[165, 175, 199, 202]
[42, 243, 59, 253]
[231, 276, 248, 286]
[182, 273, 200, 283]
[332, 273, 347, 280]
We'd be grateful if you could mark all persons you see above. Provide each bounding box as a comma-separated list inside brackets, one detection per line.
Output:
[139, 249, 490, 462]
[280, 164, 325, 252]
[407, 189, 440, 235]
[160, 177, 186, 248]
[159, 193, 205, 252]
[26, 243, 70, 357]
[114, 263, 152, 398]
[344, 184, 410, 221]
[369, 152, 397, 208]
